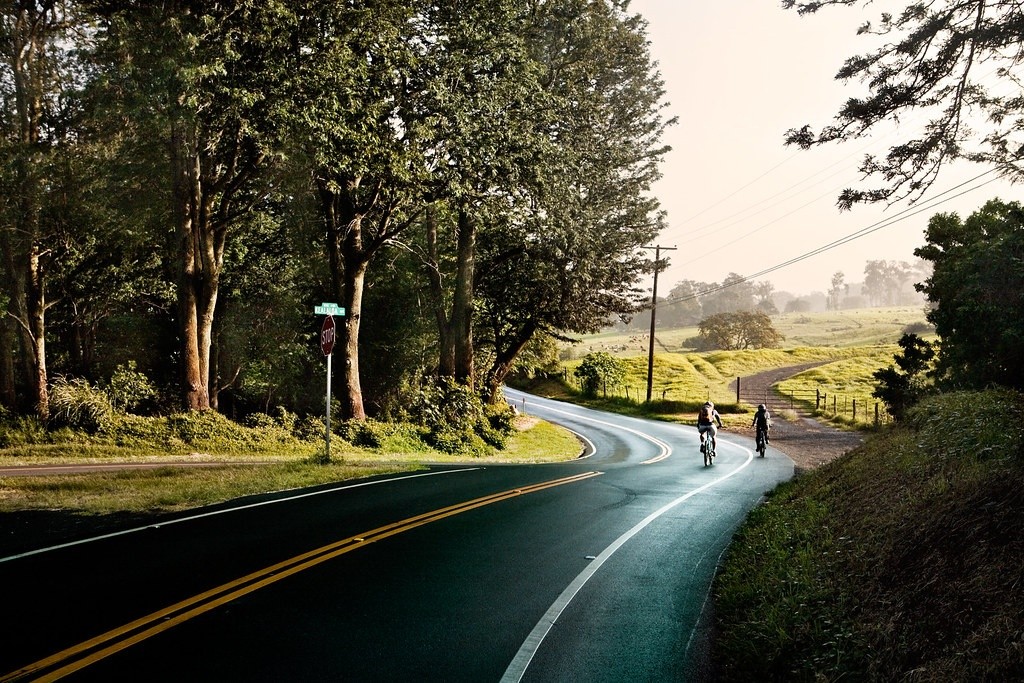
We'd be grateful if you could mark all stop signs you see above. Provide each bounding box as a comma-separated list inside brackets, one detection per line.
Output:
[320, 315, 336, 356]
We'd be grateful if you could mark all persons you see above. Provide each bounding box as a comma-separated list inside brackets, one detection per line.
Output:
[697, 401, 723, 457]
[751, 404, 771, 452]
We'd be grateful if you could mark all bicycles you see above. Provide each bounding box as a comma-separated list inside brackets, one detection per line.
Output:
[698, 425, 722, 466]
[752, 425, 772, 457]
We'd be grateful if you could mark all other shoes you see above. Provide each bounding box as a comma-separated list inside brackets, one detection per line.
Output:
[713, 451, 715, 457]
[756, 445, 761, 452]
[700, 444, 704, 452]
[766, 440, 770, 444]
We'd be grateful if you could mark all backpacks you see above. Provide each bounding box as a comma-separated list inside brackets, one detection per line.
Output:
[699, 405, 714, 425]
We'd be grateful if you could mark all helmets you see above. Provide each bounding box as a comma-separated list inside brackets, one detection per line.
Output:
[758, 404, 766, 410]
